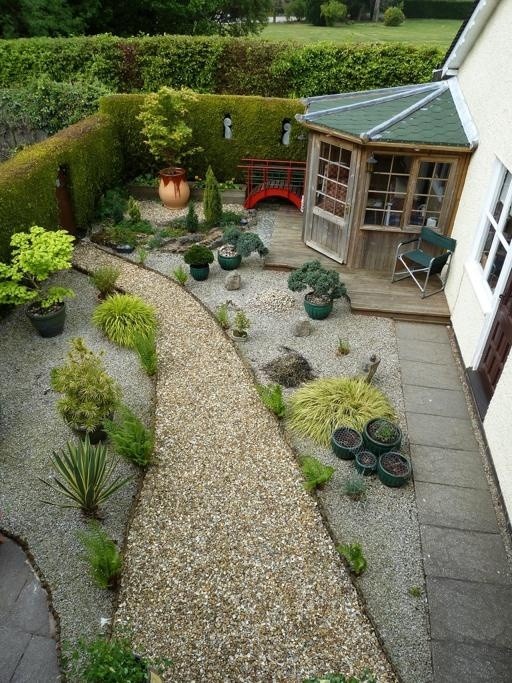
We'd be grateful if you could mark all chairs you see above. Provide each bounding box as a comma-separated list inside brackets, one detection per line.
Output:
[392, 229, 456, 297]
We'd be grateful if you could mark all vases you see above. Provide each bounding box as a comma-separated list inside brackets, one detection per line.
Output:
[332, 415, 411, 486]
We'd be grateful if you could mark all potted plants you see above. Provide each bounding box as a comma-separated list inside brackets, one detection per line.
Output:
[180, 244, 215, 278]
[137, 84, 199, 210]
[48, 339, 118, 444]
[2, 222, 77, 336]
[218, 226, 269, 268]
[287, 257, 347, 320]
[84, 261, 127, 302]
